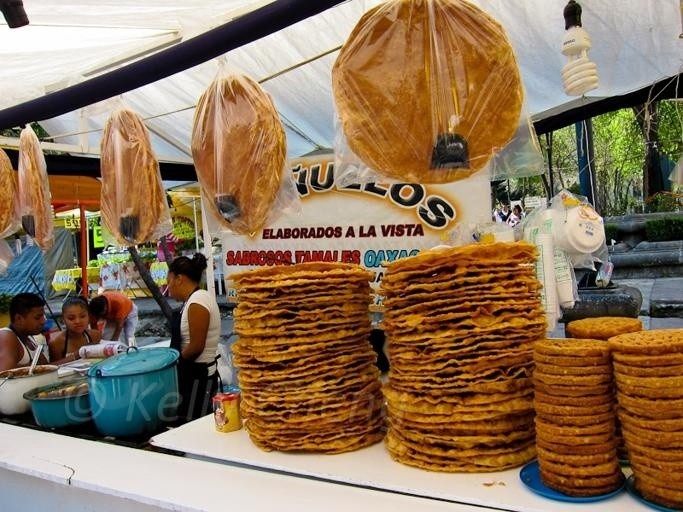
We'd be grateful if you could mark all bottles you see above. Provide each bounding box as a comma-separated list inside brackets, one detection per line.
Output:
[595, 256, 615, 288]
[78, 343, 128, 358]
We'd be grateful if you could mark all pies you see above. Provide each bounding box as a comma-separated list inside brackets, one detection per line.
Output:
[97, 112, 165, 249]
[530, 314, 683, 510]
[331, 0, 526, 183]
[226, 239, 547, 475]
[0, 128, 53, 249]
[191, 75, 283, 236]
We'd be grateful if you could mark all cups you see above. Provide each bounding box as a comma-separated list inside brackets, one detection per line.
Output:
[211, 392, 244, 433]
[427, 223, 575, 332]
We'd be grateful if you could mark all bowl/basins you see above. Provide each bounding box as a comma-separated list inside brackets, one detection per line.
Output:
[22, 377, 94, 433]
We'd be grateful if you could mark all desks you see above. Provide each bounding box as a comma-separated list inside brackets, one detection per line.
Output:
[53, 267, 99, 303]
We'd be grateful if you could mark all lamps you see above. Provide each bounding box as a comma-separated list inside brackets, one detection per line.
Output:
[558, 1, 599, 100]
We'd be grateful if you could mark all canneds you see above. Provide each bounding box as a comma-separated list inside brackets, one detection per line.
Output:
[213, 394, 242, 436]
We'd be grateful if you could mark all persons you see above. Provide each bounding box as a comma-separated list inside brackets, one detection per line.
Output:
[506, 205, 524, 228]
[492, 201, 512, 223]
[165, 253, 221, 425]
[47, 295, 100, 364]
[88, 291, 138, 352]
[0, 292, 50, 372]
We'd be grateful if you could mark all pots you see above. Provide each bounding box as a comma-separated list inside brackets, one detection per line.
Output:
[86, 346, 181, 438]
[0, 365, 61, 416]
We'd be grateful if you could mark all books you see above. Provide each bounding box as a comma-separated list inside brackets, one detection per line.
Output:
[57, 340, 119, 378]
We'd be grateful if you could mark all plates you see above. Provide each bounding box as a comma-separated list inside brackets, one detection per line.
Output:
[518, 458, 627, 505]
[616, 456, 632, 467]
[625, 474, 683, 512]
